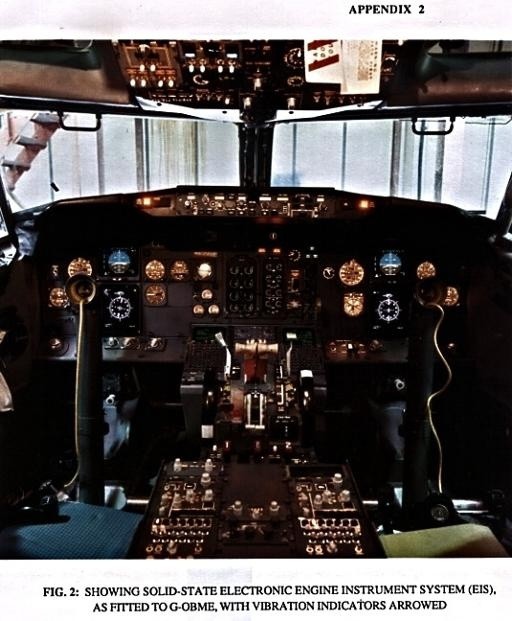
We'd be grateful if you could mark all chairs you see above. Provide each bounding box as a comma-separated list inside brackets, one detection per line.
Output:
[378, 523, 511, 557]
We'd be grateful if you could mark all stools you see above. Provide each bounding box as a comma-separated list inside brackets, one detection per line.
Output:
[0, 501, 145, 560]
